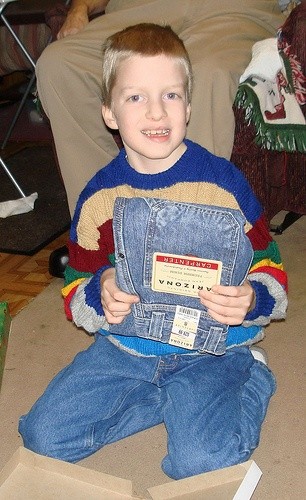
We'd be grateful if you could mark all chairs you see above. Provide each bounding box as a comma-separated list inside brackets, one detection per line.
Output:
[0, 0, 70, 219]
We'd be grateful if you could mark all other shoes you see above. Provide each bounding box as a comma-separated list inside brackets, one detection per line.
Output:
[248, 345, 268, 366]
[49, 246, 70, 278]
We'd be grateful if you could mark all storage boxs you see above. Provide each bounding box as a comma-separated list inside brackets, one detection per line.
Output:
[0, 447, 262, 500]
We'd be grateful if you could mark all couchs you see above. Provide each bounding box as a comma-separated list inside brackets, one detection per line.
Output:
[227, 0, 306, 234]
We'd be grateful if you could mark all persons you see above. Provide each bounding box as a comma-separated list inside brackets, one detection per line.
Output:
[18, 23, 289, 481]
[35, 0, 301, 279]
